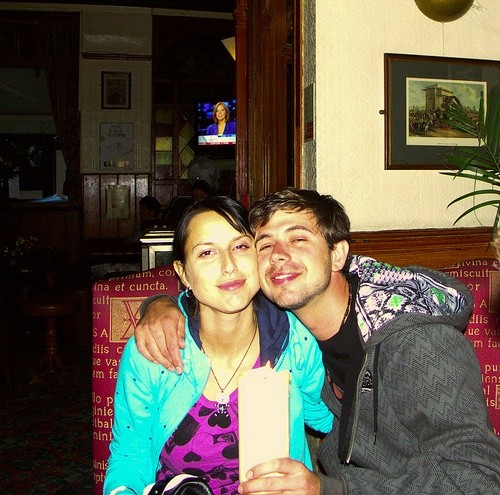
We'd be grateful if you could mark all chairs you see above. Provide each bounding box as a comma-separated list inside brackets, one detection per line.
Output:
[91, 258, 500, 495]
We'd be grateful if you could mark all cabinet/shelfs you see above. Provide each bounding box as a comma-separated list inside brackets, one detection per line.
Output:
[1, 107, 235, 274]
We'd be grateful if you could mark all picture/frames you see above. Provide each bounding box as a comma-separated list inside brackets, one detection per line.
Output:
[100, 71, 132, 110]
[383, 53, 500, 171]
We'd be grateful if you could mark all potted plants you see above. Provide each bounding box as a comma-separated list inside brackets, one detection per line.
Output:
[438, 90, 499, 256]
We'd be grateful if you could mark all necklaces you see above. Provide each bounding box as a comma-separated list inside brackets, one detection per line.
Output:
[337, 281, 352, 333]
[200, 314, 258, 417]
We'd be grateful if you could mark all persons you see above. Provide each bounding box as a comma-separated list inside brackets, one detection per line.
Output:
[103, 194, 334, 495]
[139, 179, 212, 234]
[134, 186, 500, 495]
[206, 102, 236, 136]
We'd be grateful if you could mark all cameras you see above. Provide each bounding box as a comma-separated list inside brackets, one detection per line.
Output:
[142, 474, 213, 495]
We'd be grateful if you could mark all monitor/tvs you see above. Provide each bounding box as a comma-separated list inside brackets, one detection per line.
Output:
[193, 96, 237, 154]
